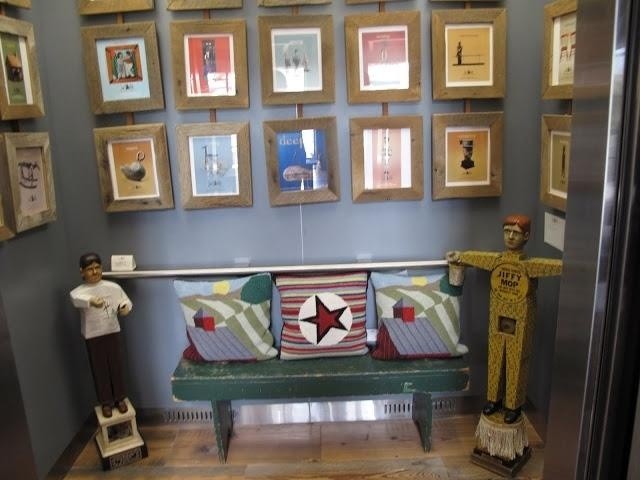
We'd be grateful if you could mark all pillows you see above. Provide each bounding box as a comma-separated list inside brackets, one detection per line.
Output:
[274, 268, 370, 361]
[369, 271, 472, 360]
[173, 273, 279, 364]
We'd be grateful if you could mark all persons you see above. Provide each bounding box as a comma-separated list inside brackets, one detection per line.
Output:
[68, 252, 133, 419]
[303, 53, 310, 69]
[285, 52, 291, 68]
[117, 52, 125, 80]
[124, 51, 134, 78]
[445, 215, 562, 425]
[457, 42, 463, 64]
[293, 49, 301, 69]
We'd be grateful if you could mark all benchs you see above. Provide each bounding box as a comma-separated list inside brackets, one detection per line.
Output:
[172, 350, 468, 464]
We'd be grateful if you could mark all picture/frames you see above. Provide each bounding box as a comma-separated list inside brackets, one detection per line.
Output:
[350, 116, 424, 203]
[431, 9, 509, 103]
[0, 18, 45, 122]
[175, 117, 253, 209]
[1, 128, 60, 240]
[77, 1, 156, 16]
[78, 22, 165, 116]
[431, 112, 504, 201]
[169, 19, 251, 109]
[258, 13, 335, 107]
[93, 123, 175, 214]
[260, 114, 340, 207]
[345, 11, 420, 106]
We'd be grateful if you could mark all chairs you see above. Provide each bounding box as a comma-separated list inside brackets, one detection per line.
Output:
[539, 114, 573, 216]
[539, 1, 577, 101]
